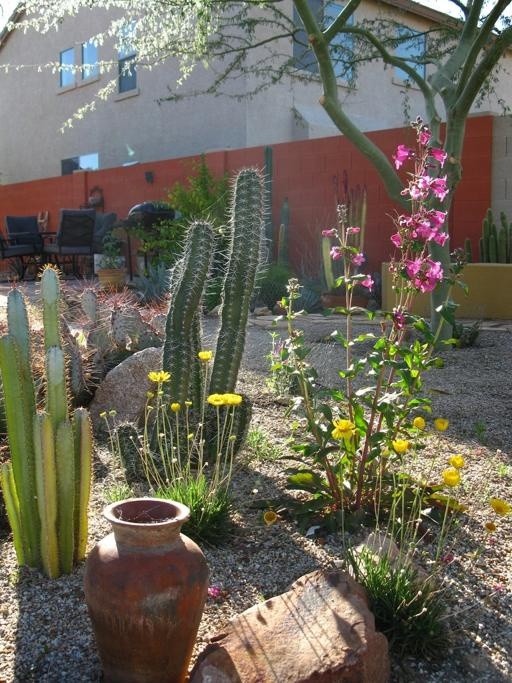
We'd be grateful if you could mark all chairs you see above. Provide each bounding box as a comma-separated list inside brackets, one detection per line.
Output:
[2, 201, 175, 283]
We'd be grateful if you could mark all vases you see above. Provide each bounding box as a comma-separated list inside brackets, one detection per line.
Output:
[86, 496, 209, 682]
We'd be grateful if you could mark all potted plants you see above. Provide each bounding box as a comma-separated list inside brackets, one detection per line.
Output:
[323, 183, 368, 308]
